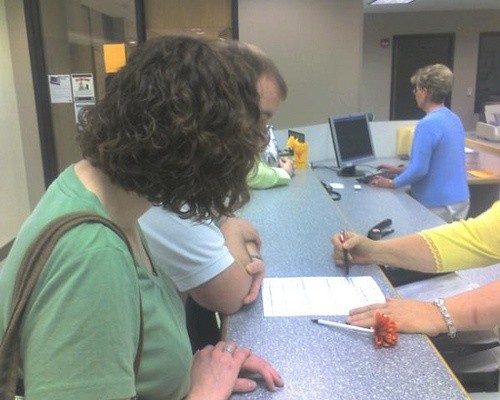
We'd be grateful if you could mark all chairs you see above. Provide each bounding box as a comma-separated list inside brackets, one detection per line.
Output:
[476, 102, 500, 142]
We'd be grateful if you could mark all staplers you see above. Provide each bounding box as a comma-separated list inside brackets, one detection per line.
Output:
[367, 218, 394, 240]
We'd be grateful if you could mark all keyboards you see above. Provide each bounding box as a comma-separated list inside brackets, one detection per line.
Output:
[355, 170, 397, 184]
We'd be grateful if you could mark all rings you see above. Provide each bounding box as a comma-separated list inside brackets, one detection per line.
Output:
[224, 345, 233, 352]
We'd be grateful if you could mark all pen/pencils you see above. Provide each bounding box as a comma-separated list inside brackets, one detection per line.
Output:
[311, 319, 375, 333]
[340, 229, 349, 280]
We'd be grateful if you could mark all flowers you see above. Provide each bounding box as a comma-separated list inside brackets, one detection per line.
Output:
[374, 312, 399, 348]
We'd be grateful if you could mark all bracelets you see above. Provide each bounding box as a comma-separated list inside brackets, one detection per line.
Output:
[433, 298, 456, 338]
[250, 256, 261, 260]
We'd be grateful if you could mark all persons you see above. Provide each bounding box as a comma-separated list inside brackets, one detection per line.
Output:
[248, 158, 295, 189]
[0, 35, 284, 400]
[333, 199, 500, 336]
[138, 43, 288, 315]
[369, 63, 470, 223]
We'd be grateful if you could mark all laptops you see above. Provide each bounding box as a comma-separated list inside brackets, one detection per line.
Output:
[328, 112, 404, 171]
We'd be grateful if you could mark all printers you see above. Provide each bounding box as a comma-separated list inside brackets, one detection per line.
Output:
[476, 102, 500, 141]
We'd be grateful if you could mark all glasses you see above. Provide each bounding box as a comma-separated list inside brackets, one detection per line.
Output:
[413, 88, 424, 95]
